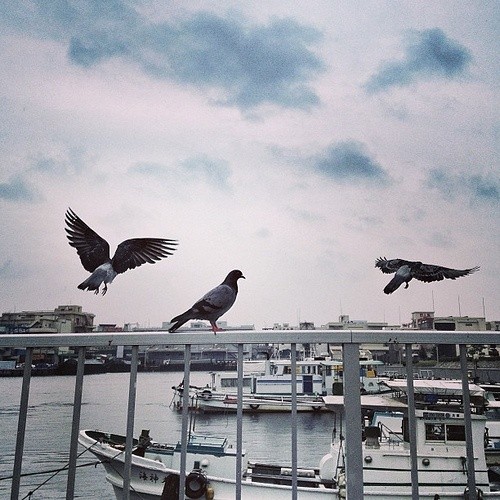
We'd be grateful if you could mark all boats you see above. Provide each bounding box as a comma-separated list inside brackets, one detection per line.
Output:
[78, 380, 500, 500]
[172, 361, 386, 413]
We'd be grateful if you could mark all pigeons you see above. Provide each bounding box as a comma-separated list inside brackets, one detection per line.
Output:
[375, 256, 481, 296]
[169, 270, 245, 335]
[63, 207, 180, 296]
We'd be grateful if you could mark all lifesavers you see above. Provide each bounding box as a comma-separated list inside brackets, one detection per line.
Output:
[201, 390, 212, 401]
[184, 472, 207, 499]
